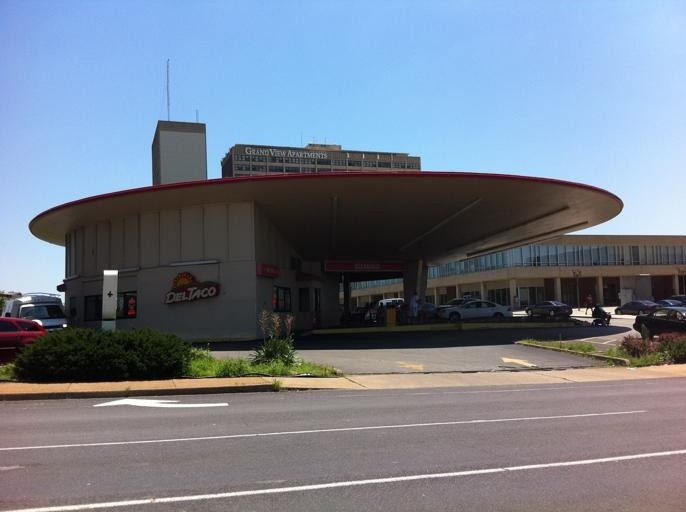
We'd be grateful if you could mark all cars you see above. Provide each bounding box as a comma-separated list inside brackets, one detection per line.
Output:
[614, 298, 685, 338]
[525, 301, 573, 318]
[433, 297, 514, 322]
[1, 316, 51, 359]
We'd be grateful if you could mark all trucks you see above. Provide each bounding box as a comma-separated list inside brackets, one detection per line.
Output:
[2, 295, 68, 331]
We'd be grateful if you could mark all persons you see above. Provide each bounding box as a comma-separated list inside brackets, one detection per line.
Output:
[594, 303, 611, 327]
[585, 294, 593, 314]
[409, 292, 422, 324]
